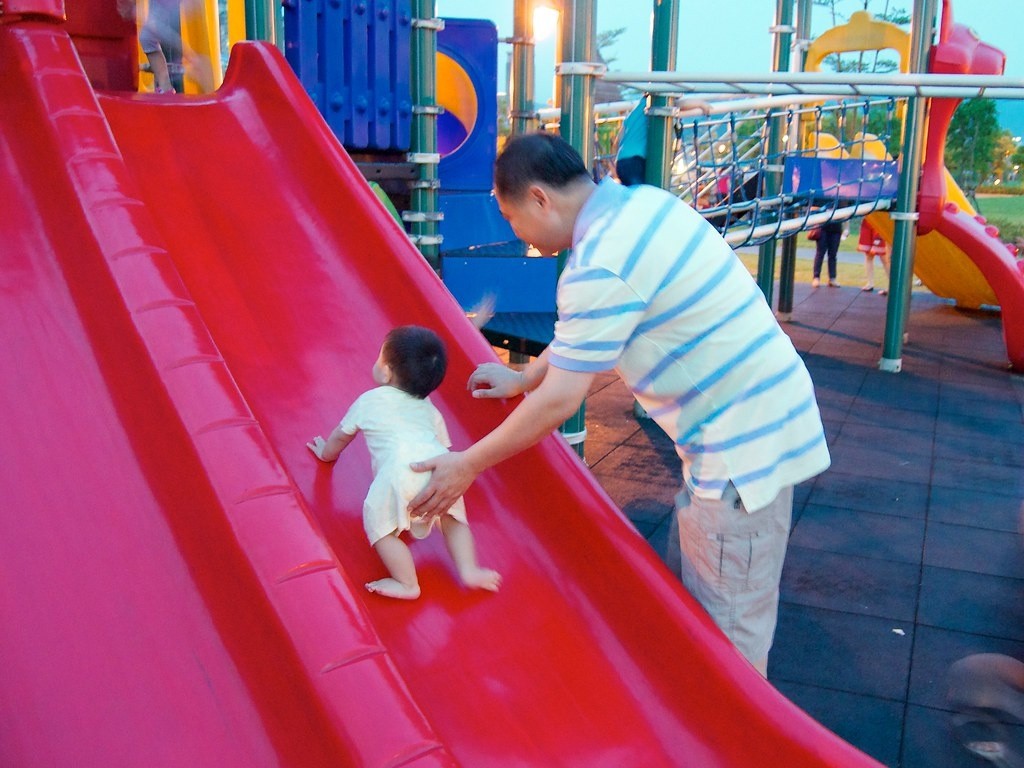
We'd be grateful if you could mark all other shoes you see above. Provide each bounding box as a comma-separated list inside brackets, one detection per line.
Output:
[863, 285, 874, 291]
[812, 279, 820, 288]
[828, 280, 840, 288]
[878, 289, 888, 295]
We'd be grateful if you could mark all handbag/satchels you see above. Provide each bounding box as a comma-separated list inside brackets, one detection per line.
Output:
[808, 225, 821, 241]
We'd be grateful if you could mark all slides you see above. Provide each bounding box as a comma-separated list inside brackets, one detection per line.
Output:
[2, 19, 889, 768]
[809, 130, 1000, 311]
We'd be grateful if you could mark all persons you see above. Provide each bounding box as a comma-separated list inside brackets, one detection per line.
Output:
[857, 217, 892, 296]
[613, 77, 714, 188]
[407, 130, 833, 679]
[688, 164, 733, 234]
[138, 0, 215, 96]
[307, 327, 503, 599]
[812, 199, 851, 289]
